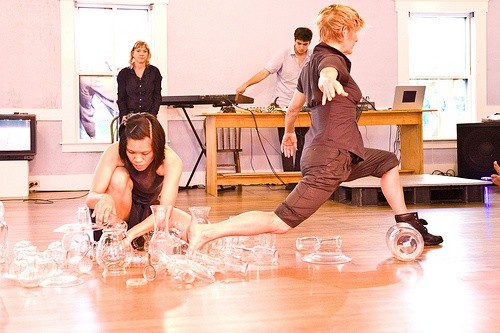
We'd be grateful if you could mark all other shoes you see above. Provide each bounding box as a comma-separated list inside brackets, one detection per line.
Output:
[286, 183, 298, 190]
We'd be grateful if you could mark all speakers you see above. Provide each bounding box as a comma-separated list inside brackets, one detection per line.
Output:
[456, 123, 500, 181]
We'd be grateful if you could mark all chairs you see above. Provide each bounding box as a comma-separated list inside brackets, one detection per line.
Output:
[204, 117, 243, 196]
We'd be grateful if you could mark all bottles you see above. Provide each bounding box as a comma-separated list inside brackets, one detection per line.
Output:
[0, 205, 279, 287]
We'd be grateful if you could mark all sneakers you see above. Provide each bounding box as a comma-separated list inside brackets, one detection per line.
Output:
[394, 212, 443, 246]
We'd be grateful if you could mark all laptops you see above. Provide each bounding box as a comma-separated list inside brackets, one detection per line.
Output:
[384, 85, 426, 111]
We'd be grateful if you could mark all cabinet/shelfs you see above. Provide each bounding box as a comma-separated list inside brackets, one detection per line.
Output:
[0, 160, 30, 202]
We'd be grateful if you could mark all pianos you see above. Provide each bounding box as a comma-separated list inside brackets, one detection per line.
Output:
[161, 92, 255, 192]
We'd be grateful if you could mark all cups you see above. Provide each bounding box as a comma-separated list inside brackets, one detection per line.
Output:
[142, 264, 167, 281]
[295, 235, 342, 258]
[69, 256, 93, 273]
[96, 211, 127, 233]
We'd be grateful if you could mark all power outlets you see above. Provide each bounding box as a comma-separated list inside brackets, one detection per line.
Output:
[29, 180, 40, 188]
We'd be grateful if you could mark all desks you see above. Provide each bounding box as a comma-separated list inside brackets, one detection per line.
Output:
[194, 110, 438, 196]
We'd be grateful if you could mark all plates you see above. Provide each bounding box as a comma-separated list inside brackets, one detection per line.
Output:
[38, 275, 84, 287]
[174, 258, 216, 284]
[300, 253, 351, 264]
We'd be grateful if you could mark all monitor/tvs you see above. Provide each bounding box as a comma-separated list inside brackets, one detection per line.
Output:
[0, 114, 37, 160]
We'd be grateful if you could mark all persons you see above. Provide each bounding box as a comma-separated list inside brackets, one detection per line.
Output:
[235, 27, 313, 190]
[185, 3, 444, 257]
[84, 112, 209, 254]
[491, 161, 500, 186]
[116, 40, 163, 139]
